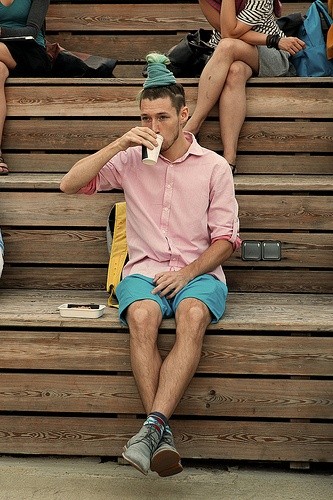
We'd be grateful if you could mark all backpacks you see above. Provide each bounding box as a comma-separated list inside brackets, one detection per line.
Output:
[289, 0, 333, 77]
[107, 201, 129, 309]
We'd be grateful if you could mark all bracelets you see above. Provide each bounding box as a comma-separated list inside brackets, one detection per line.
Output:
[266, 34, 281, 51]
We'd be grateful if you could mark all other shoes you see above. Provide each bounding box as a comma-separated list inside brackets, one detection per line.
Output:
[229, 163, 236, 177]
[0, 156, 9, 175]
[195, 130, 200, 142]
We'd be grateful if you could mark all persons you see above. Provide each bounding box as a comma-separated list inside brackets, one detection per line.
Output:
[59, 52, 244, 477]
[183, 0, 307, 176]
[0, 0, 50, 175]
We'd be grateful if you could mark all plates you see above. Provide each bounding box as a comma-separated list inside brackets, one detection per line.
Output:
[58, 303, 106, 318]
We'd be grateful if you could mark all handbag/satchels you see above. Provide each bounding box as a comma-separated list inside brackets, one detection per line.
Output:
[142, 27, 215, 78]
[46, 40, 118, 78]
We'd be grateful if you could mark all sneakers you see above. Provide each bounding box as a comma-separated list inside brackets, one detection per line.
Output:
[150, 431, 183, 477]
[121, 420, 162, 476]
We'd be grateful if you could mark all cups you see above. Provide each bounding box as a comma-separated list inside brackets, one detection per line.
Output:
[142, 134, 164, 165]
